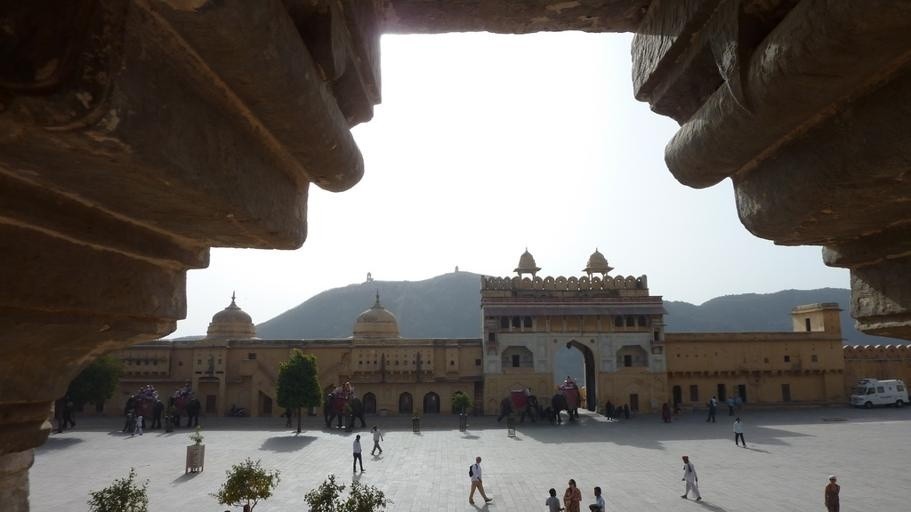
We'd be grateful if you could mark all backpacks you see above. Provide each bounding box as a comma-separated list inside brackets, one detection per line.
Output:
[469, 464, 478, 477]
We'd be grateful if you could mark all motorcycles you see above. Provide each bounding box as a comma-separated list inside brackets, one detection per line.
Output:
[228, 404, 248, 417]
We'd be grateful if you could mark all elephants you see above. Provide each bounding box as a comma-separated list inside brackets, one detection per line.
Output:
[552, 394, 574, 422]
[167, 395, 200, 428]
[122, 396, 164, 432]
[323, 397, 367, 428]
[560, 387, 580, 418]
[497, 395, 542, 422]
[55, 398, 77, 433]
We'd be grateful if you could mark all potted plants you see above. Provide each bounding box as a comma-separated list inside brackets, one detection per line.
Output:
[185, 424, 205, 474]
[452, 393, 471, 428]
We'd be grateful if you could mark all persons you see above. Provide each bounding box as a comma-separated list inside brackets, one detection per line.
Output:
[324, 381, 384, 472]
[469, 457, 492, 504]
[281, 408, 295, 428]
[680, 456, 701, 502]
[605, 400, 629, 421]
[662, 395, 743, 423]
[733, 418, 747, 448]
[497, 394, 565, 426]
[825, 475, 840, 512]
[57, 393, 201, 436]
[546, 479, 605, 512]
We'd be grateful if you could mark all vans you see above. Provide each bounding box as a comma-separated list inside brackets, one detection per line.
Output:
[848, 378, 909, 408]
